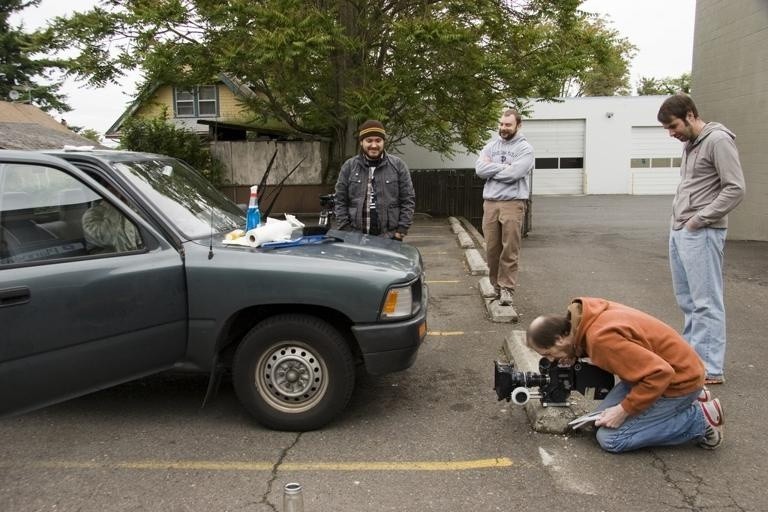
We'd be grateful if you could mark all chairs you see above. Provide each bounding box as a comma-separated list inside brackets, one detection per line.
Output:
[0, 189, 90, 261]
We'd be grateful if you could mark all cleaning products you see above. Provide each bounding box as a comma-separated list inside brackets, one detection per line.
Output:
[246, 185, 261, 231]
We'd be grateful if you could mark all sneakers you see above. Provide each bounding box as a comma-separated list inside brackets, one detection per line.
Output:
[500, 288, 513, 306]
[482, 290, 501, 301]
[691, 376, 725, 449]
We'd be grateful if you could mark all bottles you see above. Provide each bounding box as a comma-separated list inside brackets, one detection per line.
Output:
[244, 183, 263, 231]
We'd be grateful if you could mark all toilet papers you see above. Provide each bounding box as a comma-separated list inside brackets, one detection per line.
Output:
[246, 222, 291, 248]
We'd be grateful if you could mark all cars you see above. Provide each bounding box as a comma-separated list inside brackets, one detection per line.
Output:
[0, 139, 426, 437]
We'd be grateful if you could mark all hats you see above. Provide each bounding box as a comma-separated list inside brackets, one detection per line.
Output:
[359, 120, 386, 141]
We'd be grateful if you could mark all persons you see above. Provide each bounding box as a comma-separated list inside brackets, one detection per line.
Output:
[526, 298, 725, 453]
[81, 179, 143, 255]
[334, 120, 416, 241]
[475, 109, 535, 306]
[658, 94, 745, 385]
